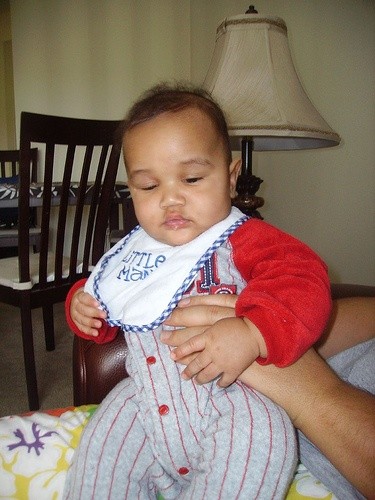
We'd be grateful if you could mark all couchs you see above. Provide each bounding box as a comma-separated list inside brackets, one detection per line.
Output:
[68, 284, 375, 413]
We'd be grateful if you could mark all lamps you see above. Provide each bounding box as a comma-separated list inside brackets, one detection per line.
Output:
[197, 4, 342, 222]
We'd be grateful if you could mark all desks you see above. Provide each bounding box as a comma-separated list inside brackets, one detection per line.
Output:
[0, 177, 135, 263]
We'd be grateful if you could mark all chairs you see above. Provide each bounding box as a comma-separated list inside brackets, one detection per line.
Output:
[1, 110, 132, 412]
[1, 146, 45, 255]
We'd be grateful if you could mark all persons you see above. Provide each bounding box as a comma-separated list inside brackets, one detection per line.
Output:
[159, 292, 374, 500]
[60, 83, 334, 499]
